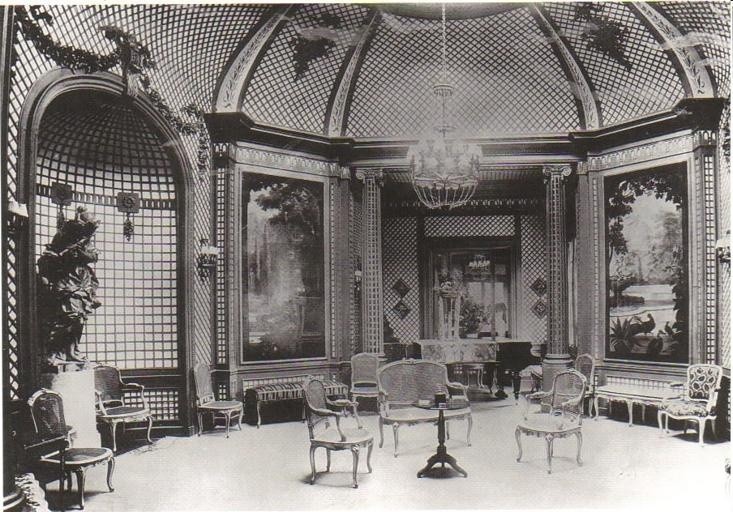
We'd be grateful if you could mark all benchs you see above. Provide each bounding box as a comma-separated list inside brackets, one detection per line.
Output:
[246, 379, 349, 428]
[594, 376, 689, 434]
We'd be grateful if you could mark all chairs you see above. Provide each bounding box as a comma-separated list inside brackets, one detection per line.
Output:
[193, 364, 243, 439]
[91, 366, 153, 452]
[657, 364, 723, 444]
[554, 353, 598, 421]
[515, 368, 587, 472]
[350, 352, 381, 417]
[375, 359, 474, 460]
[26, 389, 115, 509]
[300, 379, 375, 489]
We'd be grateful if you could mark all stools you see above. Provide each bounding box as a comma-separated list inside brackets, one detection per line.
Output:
[464, 364, 484, 388]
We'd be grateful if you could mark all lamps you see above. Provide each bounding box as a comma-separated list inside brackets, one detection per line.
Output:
[469, 254, 491, 270]
[715, 230, 731, 274]
[407, 3, 483, 210]
[199, 246, 220, 264]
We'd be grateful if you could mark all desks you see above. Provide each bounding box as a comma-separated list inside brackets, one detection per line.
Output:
[414, 339, 497, 391]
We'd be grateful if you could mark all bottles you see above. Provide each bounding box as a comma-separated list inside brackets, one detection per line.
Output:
[435, 393, 447, 408]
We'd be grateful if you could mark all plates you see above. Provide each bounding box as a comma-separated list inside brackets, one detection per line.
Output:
[413, 398, 436, 409]
